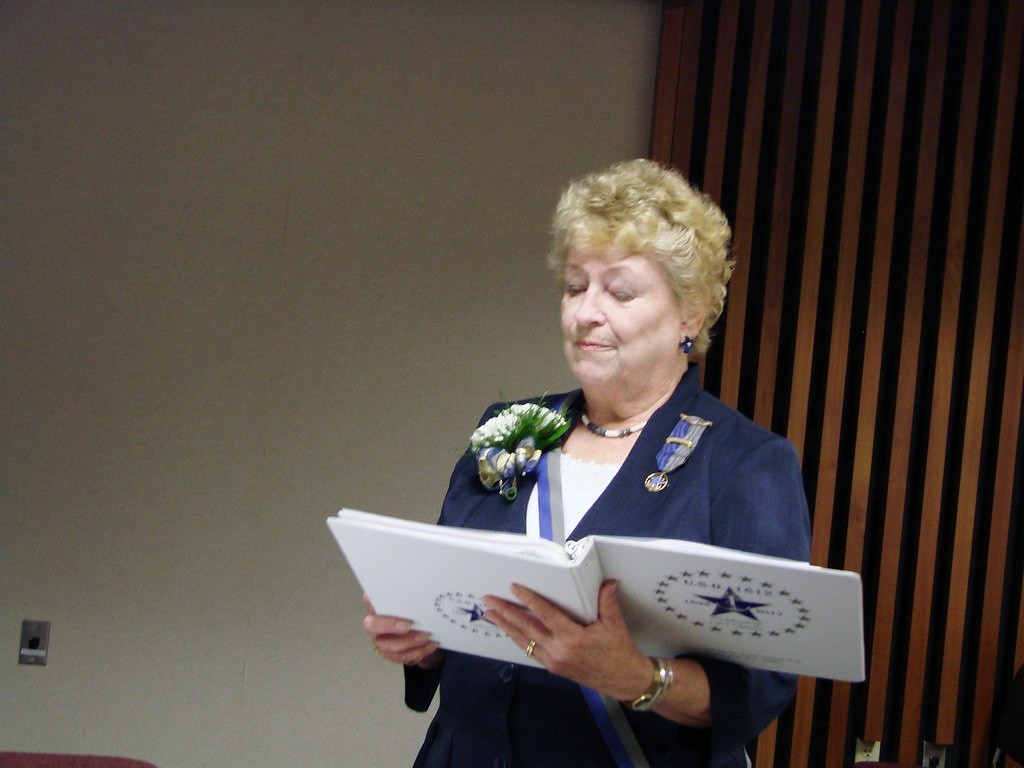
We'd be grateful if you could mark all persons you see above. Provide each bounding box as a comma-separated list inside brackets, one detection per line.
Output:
[363, 157, 812, 768]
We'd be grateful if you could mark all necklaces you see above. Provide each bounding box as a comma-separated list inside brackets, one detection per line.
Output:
[580, 413, 646, 438]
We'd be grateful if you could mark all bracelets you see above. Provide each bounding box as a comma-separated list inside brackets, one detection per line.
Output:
[619, 656, 673, 712]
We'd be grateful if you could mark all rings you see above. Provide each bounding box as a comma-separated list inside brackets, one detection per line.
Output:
[526, 640, 536, 657]
[372, 637, 383, 656]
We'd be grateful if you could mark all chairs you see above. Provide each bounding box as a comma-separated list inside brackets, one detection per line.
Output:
[989, 662, 1024, 768]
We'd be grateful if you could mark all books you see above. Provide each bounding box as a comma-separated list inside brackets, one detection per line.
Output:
[327, 507, 866, 683]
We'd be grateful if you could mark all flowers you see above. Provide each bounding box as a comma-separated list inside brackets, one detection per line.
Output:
[460, 389, 575, 459]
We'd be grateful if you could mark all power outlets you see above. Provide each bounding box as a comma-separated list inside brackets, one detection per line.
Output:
[17, 618, 50, 668]
[854, 738, 881, 764]
[922, 741, 946, 768]
